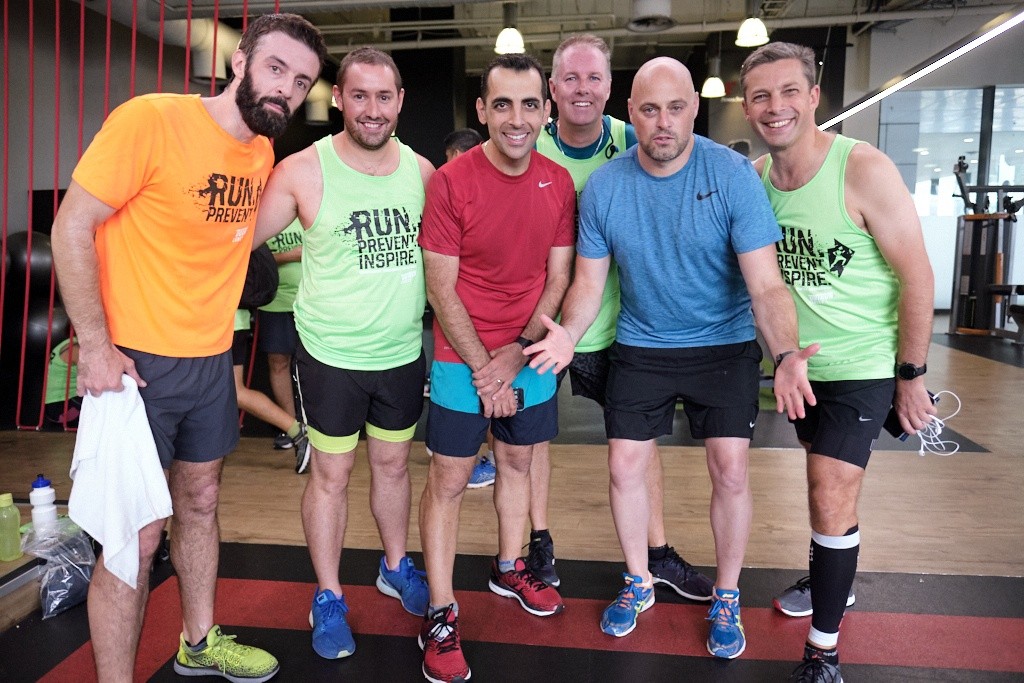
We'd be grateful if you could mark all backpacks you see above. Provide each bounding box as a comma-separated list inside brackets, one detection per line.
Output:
[238, 242, 279, 311]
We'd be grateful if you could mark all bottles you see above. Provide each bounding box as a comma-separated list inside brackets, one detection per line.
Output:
[0, 492, 24, 562]
[29, 473, 58, 537]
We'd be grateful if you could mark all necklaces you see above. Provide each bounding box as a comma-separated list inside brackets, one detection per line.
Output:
[557, 119, 604, 191]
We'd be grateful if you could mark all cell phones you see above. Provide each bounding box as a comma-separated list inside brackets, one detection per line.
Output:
[881, 393, 936, 438]
[480, 388, 524, 413]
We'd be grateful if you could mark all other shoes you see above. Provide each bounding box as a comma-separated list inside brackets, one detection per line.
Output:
[423, 371, 431, 397]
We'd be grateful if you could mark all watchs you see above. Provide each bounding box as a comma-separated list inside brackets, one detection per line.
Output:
[895, 362, 927, 380]
[775, 351, 795, 367]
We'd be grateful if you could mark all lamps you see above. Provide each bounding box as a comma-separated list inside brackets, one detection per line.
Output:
[732, 0, 773, 50]
[699, 55, 728, 101]
[490, 1, 528, 57]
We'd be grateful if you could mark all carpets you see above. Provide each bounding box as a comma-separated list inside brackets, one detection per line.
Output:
[0, 540, 1024, 683]
[239, 368, 994, 457]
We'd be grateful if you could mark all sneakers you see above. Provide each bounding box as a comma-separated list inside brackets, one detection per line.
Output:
[273, 432, 293, 450]
[290, 426, 309, 475]
[376, 554, 432, 617]
[487, 552, 564, 616]
[522, 529, 560, 589]
[468, 455, 499, 489]
[417, 600, 471, 683]
[772, 580, 857, 617]
[787, 647, 843, 683]
[172, 623, 278, 683]
[705, 584, 745, 658]
[646, 547, 715, 600]
[308, 584, 356, 659]
[599, 570, 656, 637]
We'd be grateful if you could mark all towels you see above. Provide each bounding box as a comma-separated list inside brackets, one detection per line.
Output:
[64, 374, 175, 592]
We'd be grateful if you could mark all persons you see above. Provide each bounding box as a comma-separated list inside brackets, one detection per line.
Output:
[263, 215, 304, 448]
[444, 129, 496, 488]
[45, 318, 83, 423]
[250, 46, 437, 659]
[523, 56, 820, 659]
[740, 41, 937, 683]
[523, 32, 715, 601]
[416, 52, 578, 683]
[232, 309, 311, 473]
[51, 14, 344, 683]
